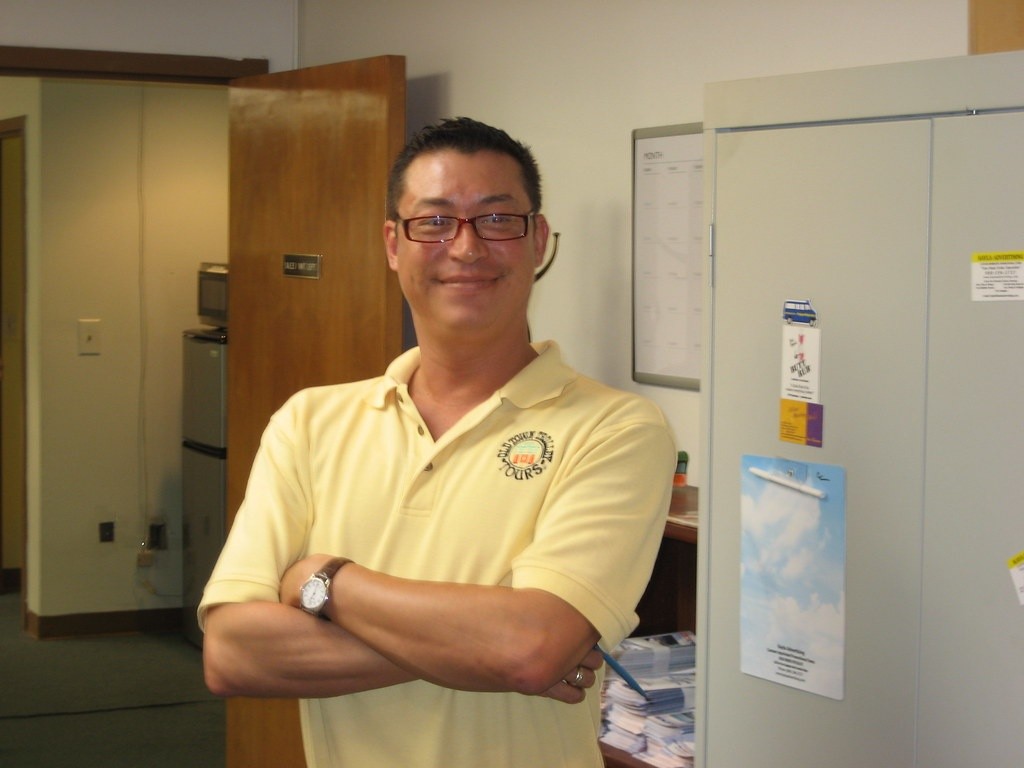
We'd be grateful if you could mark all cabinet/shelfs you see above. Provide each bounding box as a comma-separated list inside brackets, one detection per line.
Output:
[598, 484, 697, 767]
[694, 46, 1024, 768]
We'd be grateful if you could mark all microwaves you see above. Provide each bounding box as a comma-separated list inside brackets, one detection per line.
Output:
[197, 261, 229, 327]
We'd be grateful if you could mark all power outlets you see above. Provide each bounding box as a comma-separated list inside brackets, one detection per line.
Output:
[99, 522, 113, 541]
[148, 524, 168, 550]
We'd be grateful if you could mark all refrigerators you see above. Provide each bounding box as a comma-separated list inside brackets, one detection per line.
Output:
[182, 329, 227, 613]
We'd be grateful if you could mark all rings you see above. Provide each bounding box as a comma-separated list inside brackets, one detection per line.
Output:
[572, 667, 584, 686]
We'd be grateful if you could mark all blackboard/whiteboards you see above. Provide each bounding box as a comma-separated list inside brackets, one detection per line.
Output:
[631, 122, 701, 390]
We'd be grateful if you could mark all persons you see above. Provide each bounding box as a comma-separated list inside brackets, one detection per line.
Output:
[196, 113, 682, 767]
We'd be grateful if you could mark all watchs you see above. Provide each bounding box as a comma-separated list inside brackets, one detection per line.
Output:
[297, 556, 355, 621]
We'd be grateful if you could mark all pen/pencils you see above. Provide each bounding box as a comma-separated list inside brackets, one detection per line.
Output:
[747, 466, 827, 500]
[594, 642, 653, 702]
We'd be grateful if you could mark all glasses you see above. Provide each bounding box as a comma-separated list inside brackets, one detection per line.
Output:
[394, 209, 537, 243]
